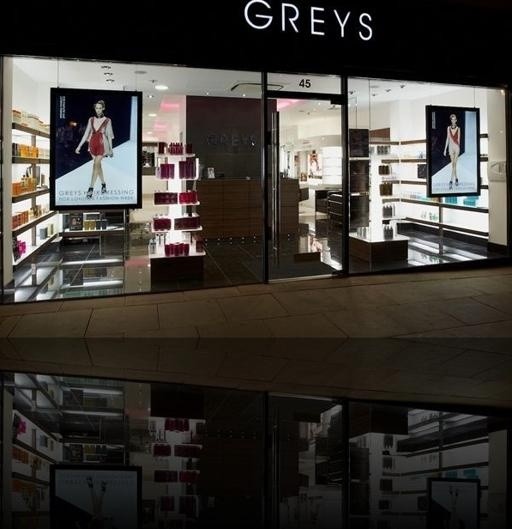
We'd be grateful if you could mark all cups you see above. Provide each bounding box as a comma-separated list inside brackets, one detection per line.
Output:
[84, 220, 108, 231]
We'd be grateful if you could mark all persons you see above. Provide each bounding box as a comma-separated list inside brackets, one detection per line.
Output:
[74, 99, 114, 200]
[447, 490, 458, 525]
[84, 474, 108, 519]
[443, 114, 460, 190]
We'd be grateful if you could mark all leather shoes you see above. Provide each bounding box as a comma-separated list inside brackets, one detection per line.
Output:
[87, 187, 93, 198]
[455, 179, 459, 186]
[449, 181, 452, 190]
[101, 183, 107, 194]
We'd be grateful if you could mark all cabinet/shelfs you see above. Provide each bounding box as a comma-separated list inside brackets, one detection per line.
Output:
[266, 120, 489, 293]
[12, 121, 266, 294]
[1, 370, 512, 528]
